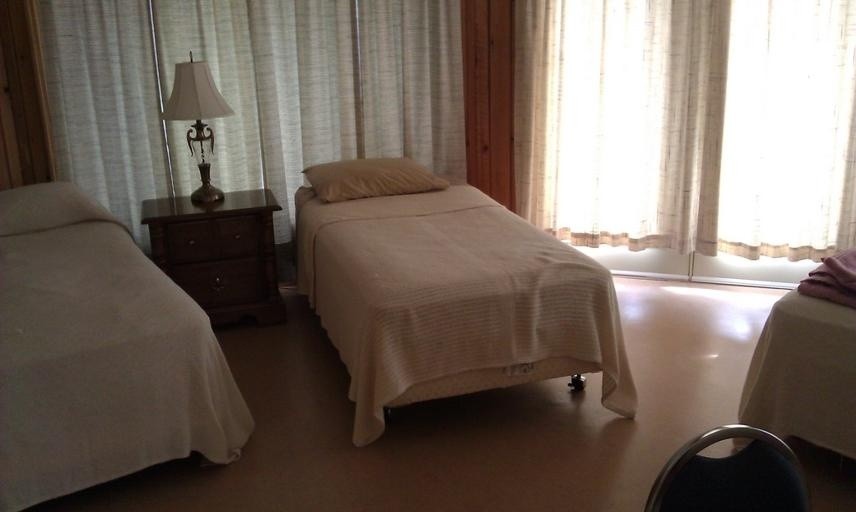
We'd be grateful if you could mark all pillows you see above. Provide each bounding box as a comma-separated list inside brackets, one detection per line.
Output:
[0, 181, 112, 237]
[301, 157, 451, 203]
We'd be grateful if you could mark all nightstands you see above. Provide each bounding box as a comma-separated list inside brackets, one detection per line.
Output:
[141, 189, 287, 327]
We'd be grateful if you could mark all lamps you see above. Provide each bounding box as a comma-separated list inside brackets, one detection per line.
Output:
[161, 51, 234, 202]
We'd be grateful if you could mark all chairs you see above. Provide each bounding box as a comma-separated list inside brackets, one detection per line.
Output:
[644, 424, 812, 512]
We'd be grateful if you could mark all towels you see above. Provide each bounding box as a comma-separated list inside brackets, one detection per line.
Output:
[797, 245, 856, 307]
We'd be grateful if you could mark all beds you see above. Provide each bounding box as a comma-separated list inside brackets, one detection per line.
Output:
[0, 220, 255, 511]
[739, 287, 856, 470]
[296, 175, 638, 448]
